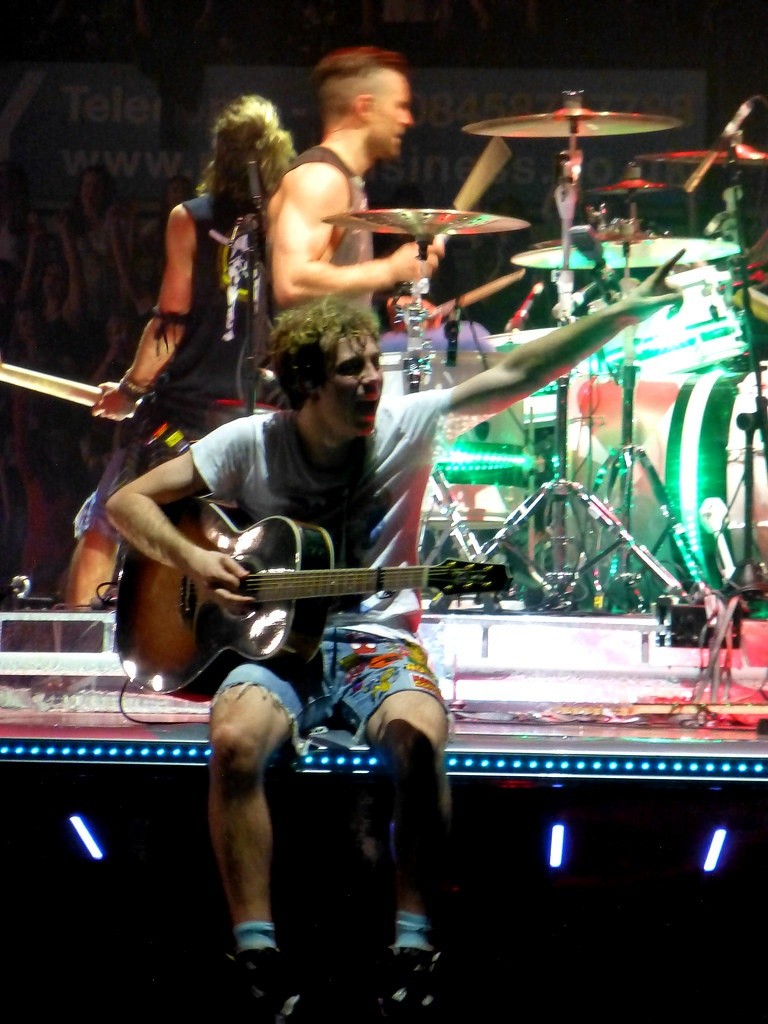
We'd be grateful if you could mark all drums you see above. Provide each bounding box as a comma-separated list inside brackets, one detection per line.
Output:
[660, 367, 767, 610]
[376, 353, 525, 470]
[483, 328, 559, 430]
[585, 265, 749, 376]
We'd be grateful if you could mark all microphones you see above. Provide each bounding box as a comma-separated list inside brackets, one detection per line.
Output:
[681, 97, 758, 192]
[248, 160, 262, 210]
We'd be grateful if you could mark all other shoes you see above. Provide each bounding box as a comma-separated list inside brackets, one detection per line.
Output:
[235, 948, 302, 1024]
[377, 944, 440, 1018]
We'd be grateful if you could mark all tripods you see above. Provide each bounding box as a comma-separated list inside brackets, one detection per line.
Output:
[428, 120, 708, 608]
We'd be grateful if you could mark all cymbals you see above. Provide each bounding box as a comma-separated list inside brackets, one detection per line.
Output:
[583, 179, 673, 195]
[319, 207, 534, 237]
[635, 144, 767, 168]
[509, 234, 743, 271]
[462, 90, 688, 139]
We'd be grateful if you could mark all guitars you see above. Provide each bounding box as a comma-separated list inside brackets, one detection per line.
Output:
[108, 488, 517, 705]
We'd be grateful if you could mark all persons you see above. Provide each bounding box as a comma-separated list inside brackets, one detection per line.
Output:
[256, 46, 445, 409]
[106, 249, 687, 1024]
[373, 184, 631, 335]
[65, 94, 298, 612]
[0, 0, 540, 69]
[0, 142, 199, 609]
